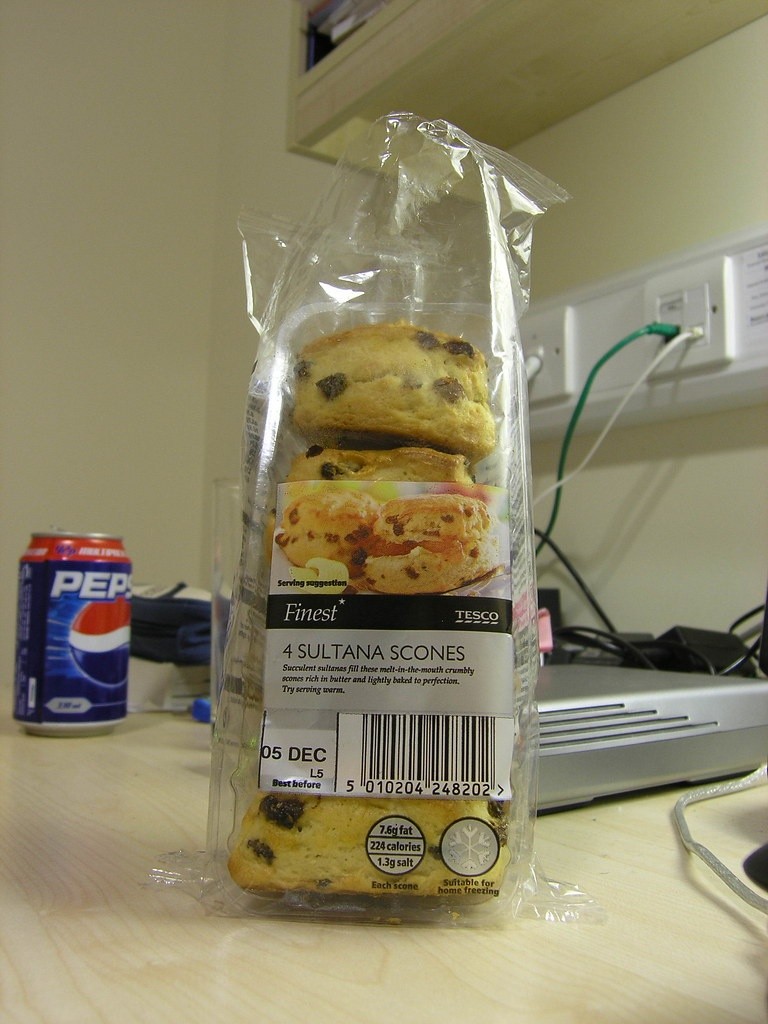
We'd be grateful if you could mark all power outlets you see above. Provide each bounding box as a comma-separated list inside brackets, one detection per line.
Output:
[639, 256, 735, 378]
[519, 305, 574, 405]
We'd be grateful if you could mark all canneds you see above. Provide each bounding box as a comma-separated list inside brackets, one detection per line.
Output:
[11, 526, 133, 737]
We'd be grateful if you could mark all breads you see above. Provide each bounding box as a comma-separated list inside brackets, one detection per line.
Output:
[358, 494, 499, 592]
[271, 488, 379, 581]
[220, 324, 511, 902]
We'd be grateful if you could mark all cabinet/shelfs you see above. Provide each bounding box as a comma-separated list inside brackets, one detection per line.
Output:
[288, 0, 768, 199]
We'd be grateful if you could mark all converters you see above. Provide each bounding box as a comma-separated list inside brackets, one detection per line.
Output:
[655, 624, 757, 677]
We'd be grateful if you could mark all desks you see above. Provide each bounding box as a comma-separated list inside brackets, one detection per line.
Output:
[0, 645, 768, 1023]
